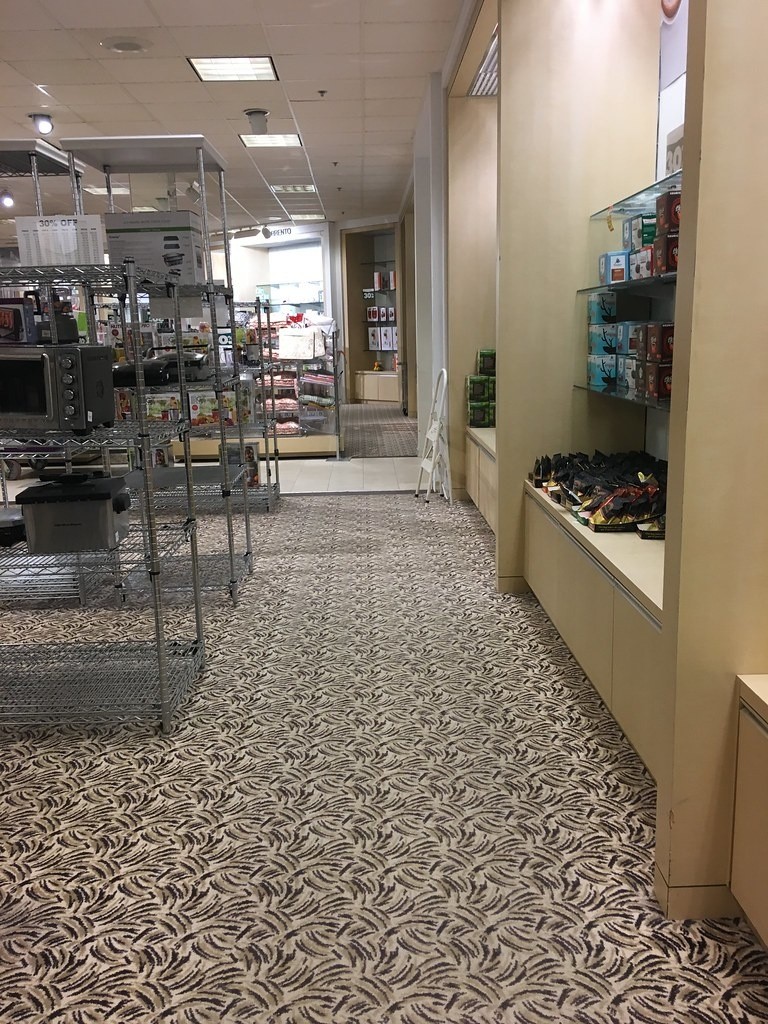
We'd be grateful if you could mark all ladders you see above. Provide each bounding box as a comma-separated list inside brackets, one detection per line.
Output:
[414, 368, 454, 509]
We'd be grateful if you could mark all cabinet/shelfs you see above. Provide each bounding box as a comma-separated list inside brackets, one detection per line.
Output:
[521, 479, 665, 786]
[0, 132, 340, 740]
[351, 259, 399, 406]
[572, 169, 685, 416]
[462, 424, 499, 540]
[723, 674, 768, 950]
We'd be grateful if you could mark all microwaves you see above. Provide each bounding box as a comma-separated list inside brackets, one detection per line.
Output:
[0, 344, 115, 438]
